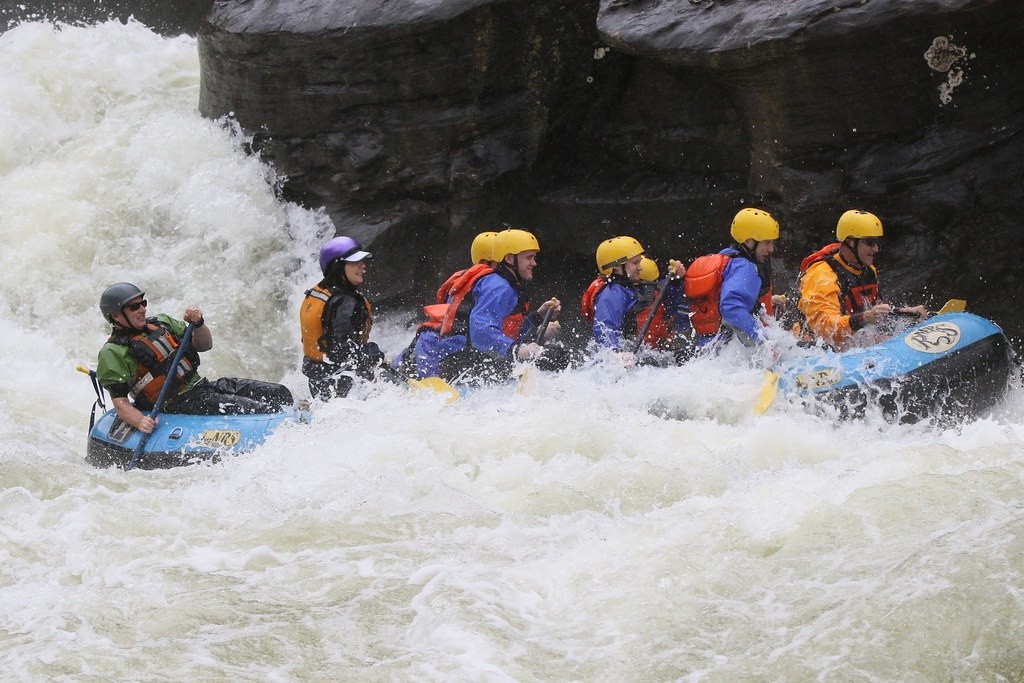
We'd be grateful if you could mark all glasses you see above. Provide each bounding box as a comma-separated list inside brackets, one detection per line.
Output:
[850, 236, 883, 246]
[122, 298, 148, 310]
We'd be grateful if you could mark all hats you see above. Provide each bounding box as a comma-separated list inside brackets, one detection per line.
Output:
[344, 249, 373, 261]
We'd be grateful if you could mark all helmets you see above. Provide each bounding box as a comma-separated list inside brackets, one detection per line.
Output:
[492, 229, 540, 262]
[99, 281, 146, 323]
[320, 237, 362, 274]
[595, 235, 645, 276]
[470, 231, 500, 265]
[639, 258, 659, 281]
[836, 210, 884, 242]
[730, 209, 780, 243]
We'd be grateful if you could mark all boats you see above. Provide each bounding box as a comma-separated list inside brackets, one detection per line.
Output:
[88, 312, 1010, 467]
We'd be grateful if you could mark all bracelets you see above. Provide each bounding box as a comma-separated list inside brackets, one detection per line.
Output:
[187, 316, 205, 329]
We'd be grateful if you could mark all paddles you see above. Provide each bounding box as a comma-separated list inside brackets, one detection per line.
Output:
[629, 263, 674, 358]
[516, 306, 554, 394]
[747, 305, 777, 408]
[884, 299, 969, 320]
[378, 361, 461, 406]
[127, 324, 194, 467]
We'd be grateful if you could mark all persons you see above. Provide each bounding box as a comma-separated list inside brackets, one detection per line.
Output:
[680, 208, 780, 351]
[300, 236, 384, 402]
[593, 235, 690, 356]
[431, 232, 565, 363]
[794, 209, 927, 349]
[416, 229, 561, 385]
[635, 258, 674, 349]
[95, 281, 294, 434]
